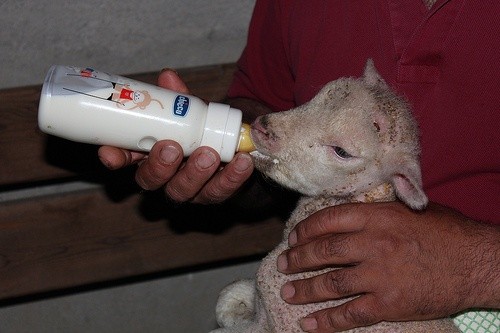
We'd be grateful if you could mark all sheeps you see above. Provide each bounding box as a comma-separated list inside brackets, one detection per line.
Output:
[212, 58, 465, 333]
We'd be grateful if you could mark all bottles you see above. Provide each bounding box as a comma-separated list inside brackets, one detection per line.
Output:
[38, 65, 257, 162]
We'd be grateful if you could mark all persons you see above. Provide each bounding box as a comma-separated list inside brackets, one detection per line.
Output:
[97, 0, 500, 333]
